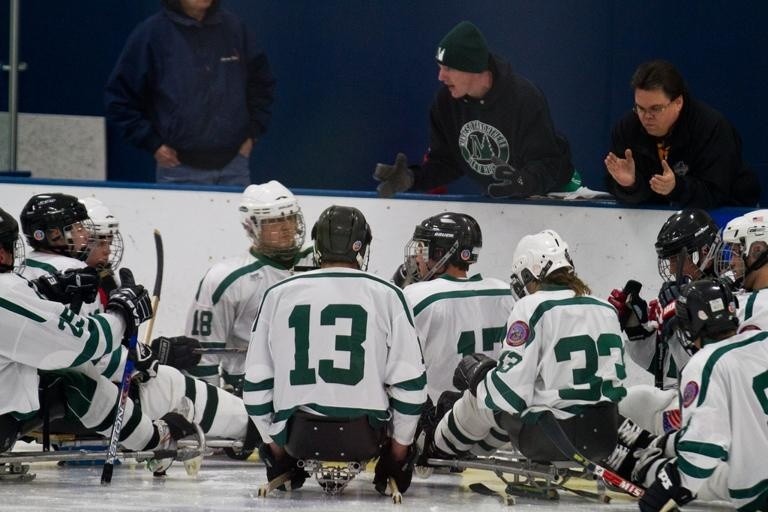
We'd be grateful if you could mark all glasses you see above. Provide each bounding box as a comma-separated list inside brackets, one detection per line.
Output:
[632, 93, 681, 115]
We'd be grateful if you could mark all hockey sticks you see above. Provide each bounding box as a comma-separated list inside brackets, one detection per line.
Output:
[1, 417, 211, 469]
[537, 409, 648, 498]
[142, 228, 166, 345]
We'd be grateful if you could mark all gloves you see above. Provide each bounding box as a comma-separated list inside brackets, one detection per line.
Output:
[607, 279, 653, 341]
[373, 455, 414, 498]
[151, 334, 202, 368]
[487, 159, 547, 197]
[639, 462, 683, 512]
[660, 280, 682, 336]
[453, 351, 496, 396]
[372, 153, 416, 198]
[128, 342, 159, 384]
[105, 267, 154, 338]
[259, 444, 303, 491]
[37, 265, 99, 305]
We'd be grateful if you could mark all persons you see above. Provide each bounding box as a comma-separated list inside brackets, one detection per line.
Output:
[618, 209, 768, 436]
[100, 0, 275, 188]
[185, 180, 315, 463]
[389, 212, 518, 473]
[601, 277, 767, 512]
[0, 204, 196, 478]
[373, 20, 580, 200]
[243, 203, 427, 503]
[411, 229, 625, 470]
[74, 196, 201, 370]
[605, 208, 740, 387]
[17, 192, 258, 461]
[601, 59, 758, 209]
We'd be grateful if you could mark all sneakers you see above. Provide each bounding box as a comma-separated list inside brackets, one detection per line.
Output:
[437, 391, 471, 473]
[139, 394, 195, 476]
[410, 411, 434, 465]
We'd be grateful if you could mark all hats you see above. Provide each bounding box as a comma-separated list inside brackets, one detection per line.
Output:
[434, 20, 491, 73]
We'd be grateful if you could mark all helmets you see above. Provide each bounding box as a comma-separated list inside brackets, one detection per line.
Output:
[403, 212, 481, 282]
[238, 180, 305, 258]
[654, 209, 721, 284]
[79, 215, 123, 277]
[674, 278, 738, 358]
[311, 204, 373, 271]
[0, 205, 25, 275]
[387, 265, 418, 287]
[20, 192, 96, 258]
[716, 209, 768, 291]
[510, 229, 575, 302]
